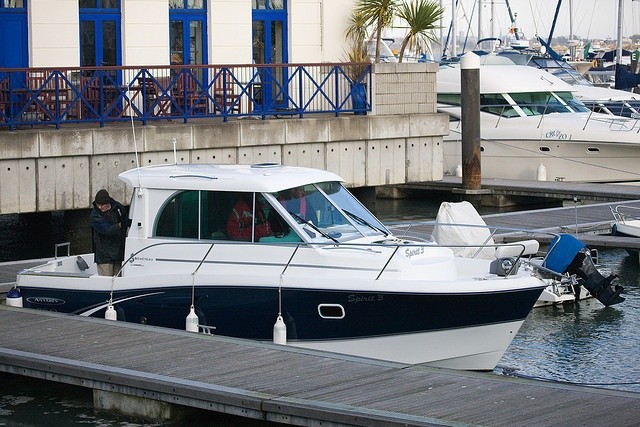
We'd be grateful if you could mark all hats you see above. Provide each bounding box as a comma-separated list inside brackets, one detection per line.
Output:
[94, 189, 111, 204]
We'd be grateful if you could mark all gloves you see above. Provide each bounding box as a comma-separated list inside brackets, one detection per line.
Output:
[120, 218, 132, 227]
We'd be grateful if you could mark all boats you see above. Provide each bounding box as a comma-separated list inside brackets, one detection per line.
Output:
[5, 160, 561, 370]
[609, 205, 640, 268]
[429, 198, 626, 309]
[560, 54, 593, 76]
[436, 54, 640, 184]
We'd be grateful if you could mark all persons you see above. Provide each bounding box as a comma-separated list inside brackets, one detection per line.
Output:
[226, 199, 272, 242]
[89, 189, 133, 276]
[267, 186, 318, 238]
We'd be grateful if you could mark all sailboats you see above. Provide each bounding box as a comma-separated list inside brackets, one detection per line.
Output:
[438, 0, 640, 120]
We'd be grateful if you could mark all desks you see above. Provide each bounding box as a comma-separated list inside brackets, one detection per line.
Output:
[226, 81, 274, 120]
[90, 85, 142, 124]
[13, 88, 72, 128]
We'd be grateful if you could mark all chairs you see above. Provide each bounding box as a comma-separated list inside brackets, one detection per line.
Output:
[48, 68, 80, 124]
[26, 70, 47, 127]
[152, 76, 184, 121]
[214, 73, 241, 115]
[81, 76, 110, 123]
[0, 77, 22, 130]
[177, 73, 208, 117]
